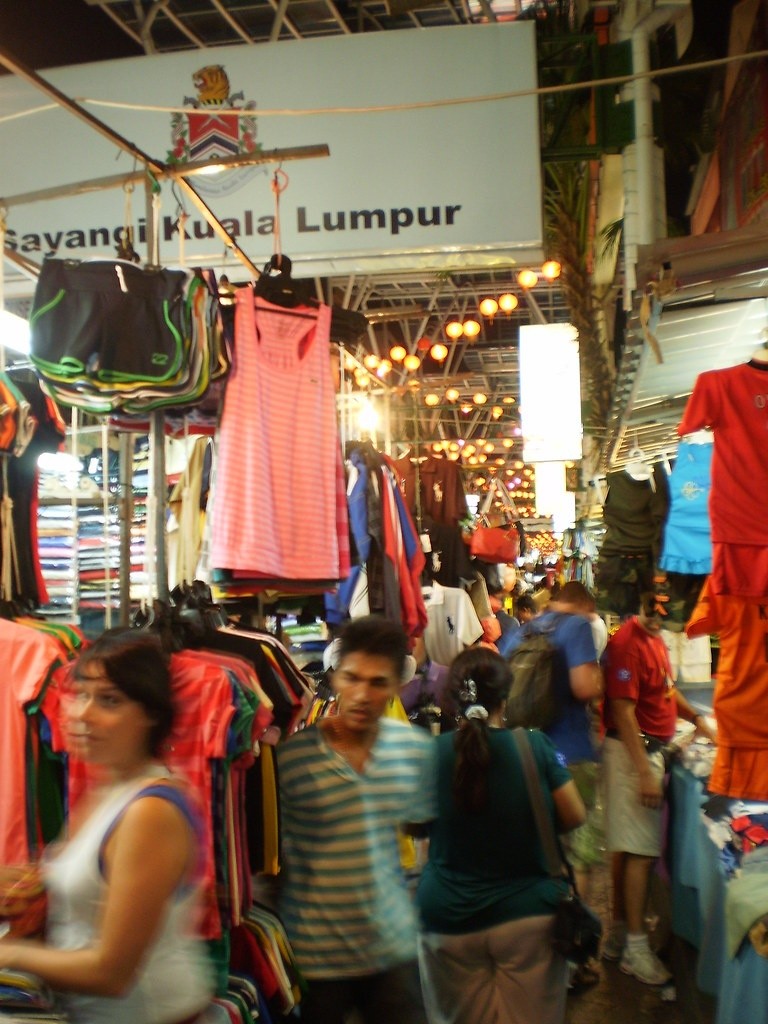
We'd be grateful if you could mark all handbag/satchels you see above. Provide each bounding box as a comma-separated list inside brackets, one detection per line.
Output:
[470, 478, 520, 564]
[551, 895, 602, 963]
[481, 479, 527, 558]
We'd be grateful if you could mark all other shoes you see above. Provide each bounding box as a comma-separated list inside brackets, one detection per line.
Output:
[598, 933, 672, 984]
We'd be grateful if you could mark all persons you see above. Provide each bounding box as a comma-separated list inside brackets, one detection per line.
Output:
[279, 614, 439, 1024]
[1, 624, 236, 1024]
[403, 567, 719, 1024]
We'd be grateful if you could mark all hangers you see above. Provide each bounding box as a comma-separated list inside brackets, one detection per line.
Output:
[0, 584, 269, 656]
[103, 241, 367, 346]
[624, 448, 653, 476]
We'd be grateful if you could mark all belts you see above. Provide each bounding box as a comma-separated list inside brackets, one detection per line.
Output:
[605, 728, 664, 752]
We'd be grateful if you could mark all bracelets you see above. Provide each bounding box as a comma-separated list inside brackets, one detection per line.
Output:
[692, 714, 703, 725]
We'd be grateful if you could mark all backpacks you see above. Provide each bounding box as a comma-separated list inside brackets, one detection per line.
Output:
[497, 612, 575, 732]
